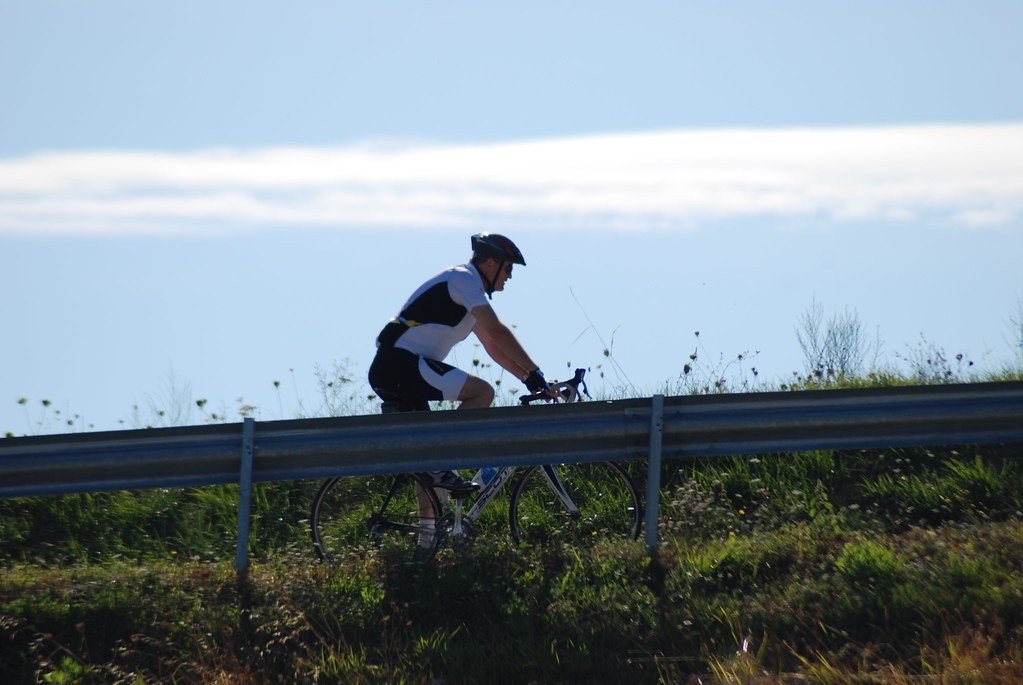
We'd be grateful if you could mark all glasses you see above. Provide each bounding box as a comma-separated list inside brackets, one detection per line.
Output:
[495, 259, 512, 273]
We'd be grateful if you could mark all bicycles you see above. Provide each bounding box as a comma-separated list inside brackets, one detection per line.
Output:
[311, 368, 643, 587]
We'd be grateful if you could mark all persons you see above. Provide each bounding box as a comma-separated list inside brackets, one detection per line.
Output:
[367, 232, 564, 567]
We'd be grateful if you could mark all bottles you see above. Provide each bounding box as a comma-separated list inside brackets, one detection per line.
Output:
[470, 467, 500, 492]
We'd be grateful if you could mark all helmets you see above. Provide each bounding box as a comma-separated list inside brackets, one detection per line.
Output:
[471, 233, 526, 266]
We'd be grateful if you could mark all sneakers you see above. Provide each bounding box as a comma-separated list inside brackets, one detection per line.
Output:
[421, 469, 481, 492]
[409, 546, 433, 563]
